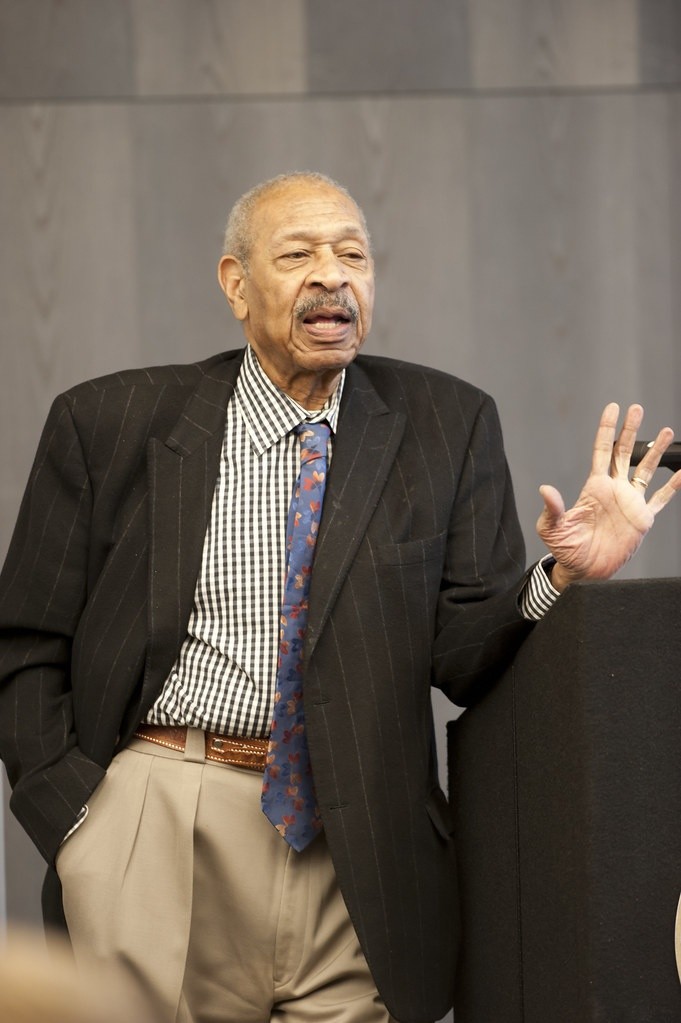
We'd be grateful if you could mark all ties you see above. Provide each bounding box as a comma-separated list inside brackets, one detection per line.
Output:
[258, 424, 324, 851]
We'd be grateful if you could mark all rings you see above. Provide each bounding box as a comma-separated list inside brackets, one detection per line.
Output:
[631, 477, 648, 489]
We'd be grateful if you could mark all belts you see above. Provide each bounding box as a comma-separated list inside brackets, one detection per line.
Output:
[132, 726, 267, 769]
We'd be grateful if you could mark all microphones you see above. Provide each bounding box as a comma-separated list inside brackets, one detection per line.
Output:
[613, 441, 681, 468]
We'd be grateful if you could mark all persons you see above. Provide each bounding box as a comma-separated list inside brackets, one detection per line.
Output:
[0, 171, 681, 1023]
